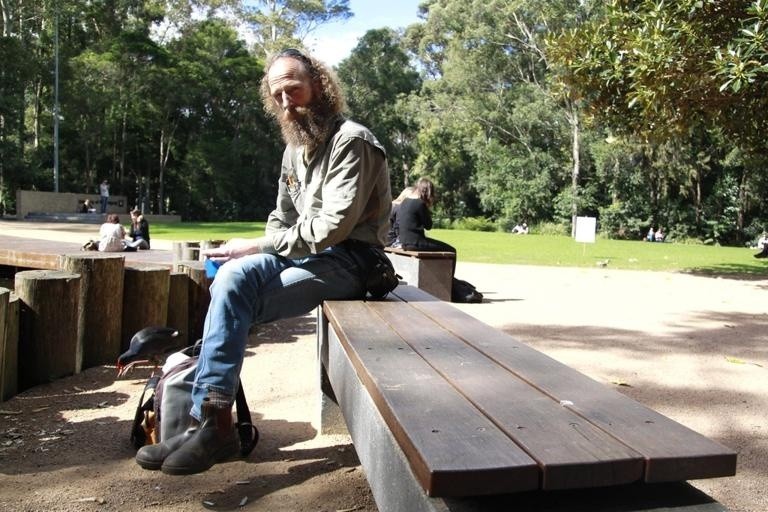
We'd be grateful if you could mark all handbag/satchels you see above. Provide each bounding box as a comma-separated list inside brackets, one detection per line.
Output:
[364, 245, 400, 300]
[130, 344, 260, 457]
[81, 239, 98, 251]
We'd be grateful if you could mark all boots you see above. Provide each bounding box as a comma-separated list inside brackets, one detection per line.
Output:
[134, 397, 249, 477]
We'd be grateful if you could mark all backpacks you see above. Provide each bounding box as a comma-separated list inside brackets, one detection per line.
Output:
[451, 276, 483, 303]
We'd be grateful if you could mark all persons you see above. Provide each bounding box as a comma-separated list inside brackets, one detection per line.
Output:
[77, 199, 93, 213]
[100, 177, 110, 213]
[758, 231, 768, 249]
[98, 209, 150, 251]
[386, 177, 483, 303]
[647, 227, 665, 242]
[136, 49, 392, 475]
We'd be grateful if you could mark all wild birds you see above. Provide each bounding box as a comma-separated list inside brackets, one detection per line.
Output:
[117, 326, 185, 378]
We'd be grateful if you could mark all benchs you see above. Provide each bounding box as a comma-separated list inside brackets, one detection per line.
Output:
[321, 244, 739, 507]
